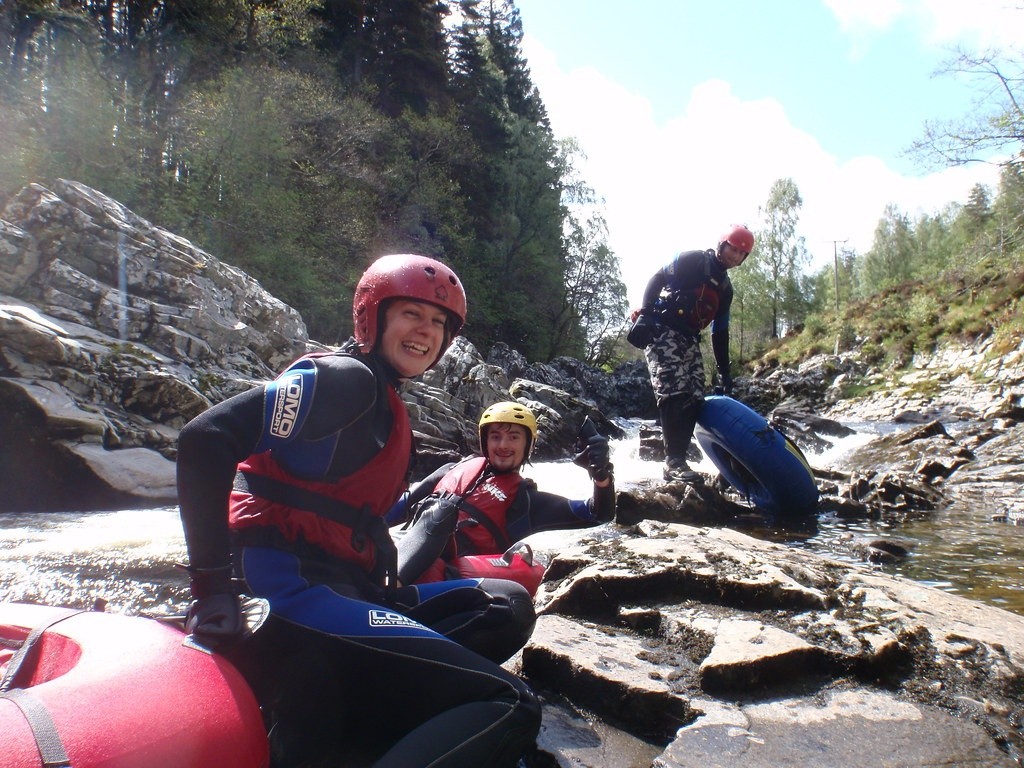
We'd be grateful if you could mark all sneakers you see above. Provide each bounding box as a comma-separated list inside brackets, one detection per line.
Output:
[664, 461, 701, 481]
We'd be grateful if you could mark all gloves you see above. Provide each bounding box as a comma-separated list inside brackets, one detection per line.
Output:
[173, 562, 243, 652]
[714, 361, 733, 395]
[630, 309, 661, 347]
[571, 414, 613, 482]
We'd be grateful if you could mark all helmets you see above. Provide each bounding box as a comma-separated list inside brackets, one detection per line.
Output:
[720, 226, 755, 267]
[353, 252, 470, 370]
[478, 401, 538, 460]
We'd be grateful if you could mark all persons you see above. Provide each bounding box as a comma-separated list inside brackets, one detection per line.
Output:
[387, 401, 615, 588]
[175, 255, 541, 768]
[627, 226, 755, 485]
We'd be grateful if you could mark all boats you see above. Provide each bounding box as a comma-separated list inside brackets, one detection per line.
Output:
[699, 396, 822, 525]
[419, 544, 546, 603]
[2, 598, 276, 765]
